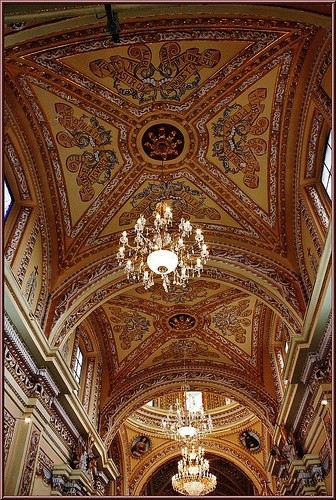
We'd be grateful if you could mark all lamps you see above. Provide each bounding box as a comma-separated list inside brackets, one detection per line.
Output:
[162, 387, 213, 442]
[115, 128, 211, 295]
[171, 442, 217, 497]
